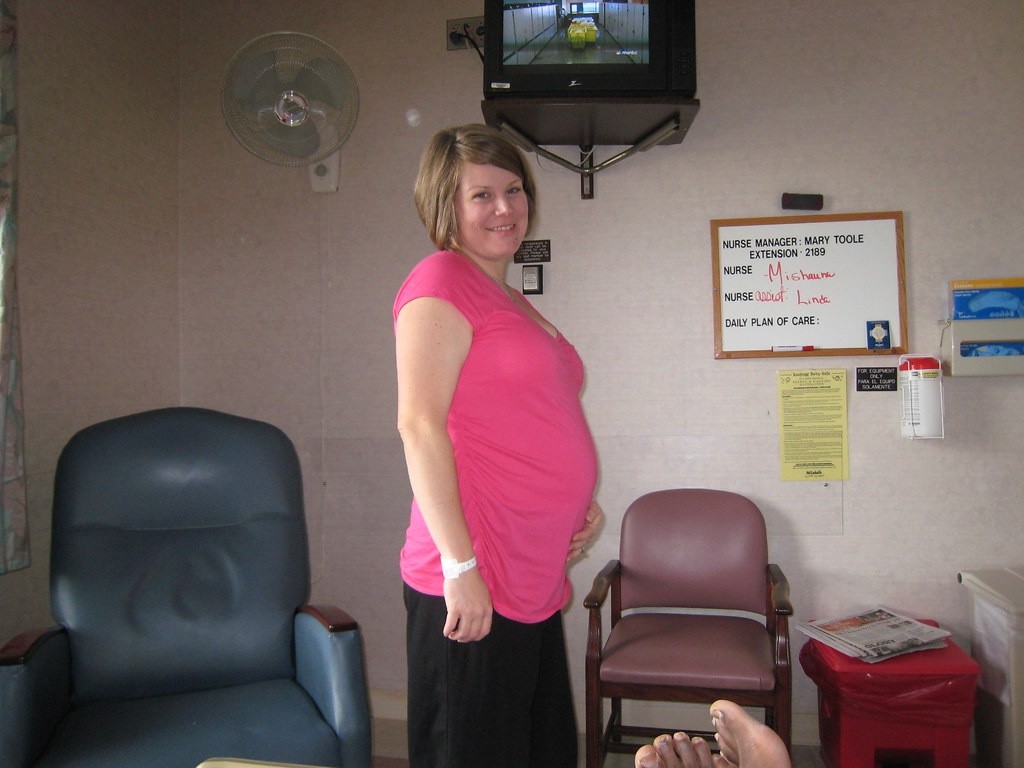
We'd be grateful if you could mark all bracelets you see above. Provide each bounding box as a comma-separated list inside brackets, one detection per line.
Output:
[438, 555, 476, 580]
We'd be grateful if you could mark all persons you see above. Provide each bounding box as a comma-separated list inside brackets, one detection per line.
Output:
[633, 699, 792, 767]
[388, 122, 607, 768]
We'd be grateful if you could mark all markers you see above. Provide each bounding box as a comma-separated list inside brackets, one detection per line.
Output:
[771, 344, 816, 354]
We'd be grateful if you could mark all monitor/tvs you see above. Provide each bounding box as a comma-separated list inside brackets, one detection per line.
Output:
[483, 0, 697, 98]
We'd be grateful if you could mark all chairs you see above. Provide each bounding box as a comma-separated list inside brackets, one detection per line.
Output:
[583, 486, 797, 768]
[2, 406, 378, 768]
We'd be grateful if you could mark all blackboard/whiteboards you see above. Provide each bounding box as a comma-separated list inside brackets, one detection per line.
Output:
[709, 209, 908, 359]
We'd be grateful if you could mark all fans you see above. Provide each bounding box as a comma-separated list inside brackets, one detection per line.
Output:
[219, 32, 363, 192]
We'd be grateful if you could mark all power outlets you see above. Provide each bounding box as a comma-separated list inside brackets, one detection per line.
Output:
[446, 15, 485, 50]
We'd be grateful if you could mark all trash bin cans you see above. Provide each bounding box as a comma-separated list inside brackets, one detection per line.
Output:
[956, 564, 1023, 768]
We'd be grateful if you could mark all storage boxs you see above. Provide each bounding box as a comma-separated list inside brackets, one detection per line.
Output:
[798, 617, 975, 768]
